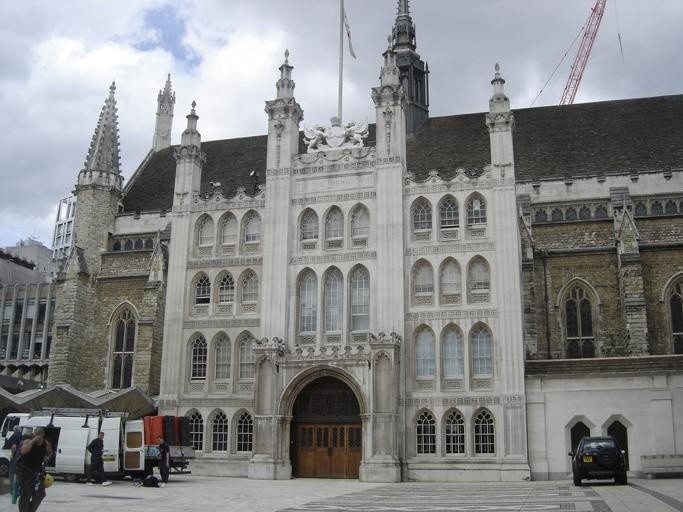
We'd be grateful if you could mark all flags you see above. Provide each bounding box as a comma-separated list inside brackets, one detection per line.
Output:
[343, 8, 357, 59]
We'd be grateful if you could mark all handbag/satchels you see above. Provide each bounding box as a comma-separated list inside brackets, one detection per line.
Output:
[143, 475, 159, 487]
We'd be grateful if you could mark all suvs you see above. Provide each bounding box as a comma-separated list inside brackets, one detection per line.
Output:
[569, 437, 627, 486]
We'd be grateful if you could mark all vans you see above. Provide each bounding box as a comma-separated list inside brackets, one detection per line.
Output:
[1, 408, 146, 482]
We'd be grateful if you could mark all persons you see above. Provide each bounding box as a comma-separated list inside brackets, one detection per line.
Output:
[156, 435, 170, 487]
[18, 427, 53, 512]
[87, 432, 112, 486]
[4, 425, 23, 497]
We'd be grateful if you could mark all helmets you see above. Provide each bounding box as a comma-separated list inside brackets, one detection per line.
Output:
[42, 474, 53, 488]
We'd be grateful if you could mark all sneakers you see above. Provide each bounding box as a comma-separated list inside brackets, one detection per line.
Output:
[157, 482, 166, 488]
[86, 482, 97, 486]
[102, 482, 113, 487]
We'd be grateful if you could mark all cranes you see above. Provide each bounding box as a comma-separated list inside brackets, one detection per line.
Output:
[528, 0, 607, 105]
